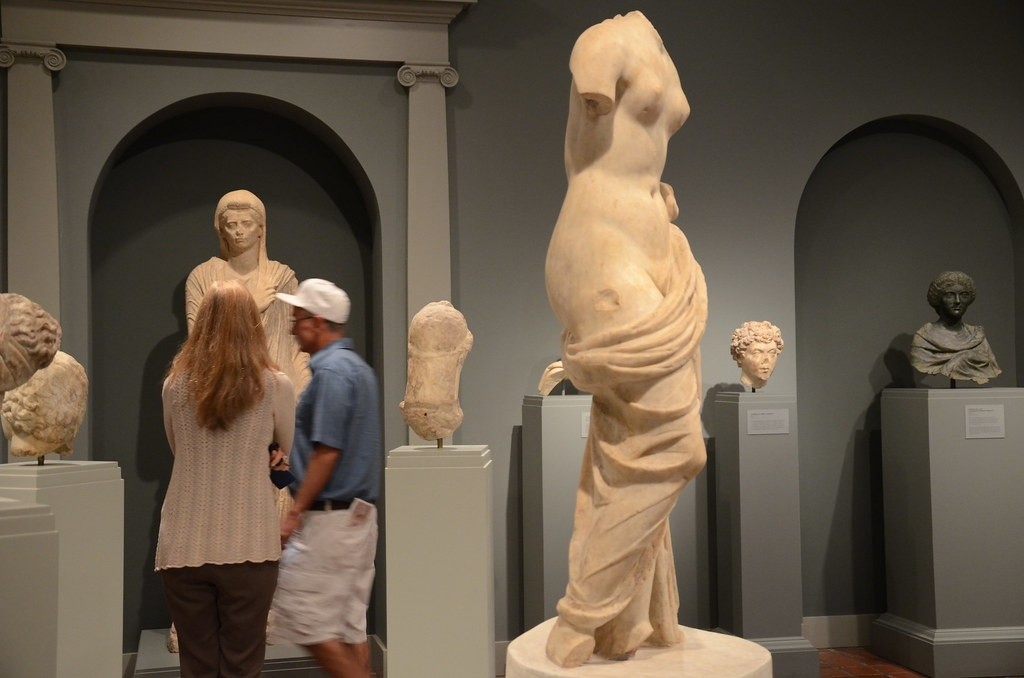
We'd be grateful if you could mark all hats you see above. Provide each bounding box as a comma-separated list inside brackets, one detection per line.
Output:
[275, 277, 351, 324]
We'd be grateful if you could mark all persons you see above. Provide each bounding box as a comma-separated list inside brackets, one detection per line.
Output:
[730, 319, 784, 388]
[910, 270, 1001, 384]
[0, 294, 88, 460]
[541, 11, 709, 668]
[153, 280, 295, 677]
[269, 278, 383, 677]
[169, 190, 312, 652]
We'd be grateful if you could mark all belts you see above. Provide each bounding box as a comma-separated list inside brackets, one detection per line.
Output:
[307, 499, 376, 512]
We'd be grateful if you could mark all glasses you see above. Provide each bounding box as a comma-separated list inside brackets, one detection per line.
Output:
[288, 315, 313, 324]
